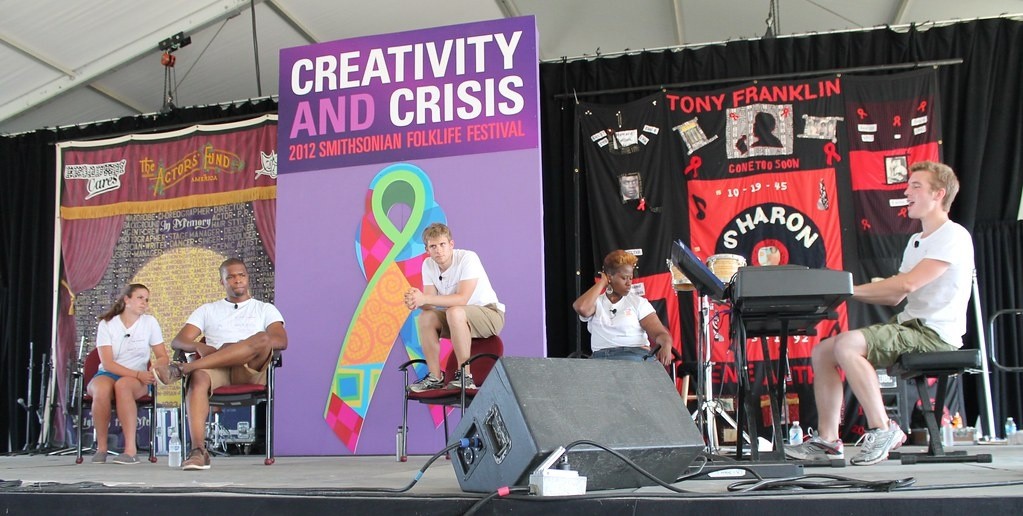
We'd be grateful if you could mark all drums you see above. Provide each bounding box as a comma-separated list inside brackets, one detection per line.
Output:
[705, 253, 747, 290]
[667, 257, 701, 292]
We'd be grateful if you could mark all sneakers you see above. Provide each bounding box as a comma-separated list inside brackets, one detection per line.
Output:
[783, 426, 844, 460]
[850, 420, 907, 465]
[183, 448, 210, 470]
[152, 361, 184, 386]
[406, 371, 445, 392]
[447, 371, 477, 389]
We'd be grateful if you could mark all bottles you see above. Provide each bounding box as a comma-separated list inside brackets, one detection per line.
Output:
[1005, 417, 1016, 439]
[789, 421, 803, 445]
[168, 433, 181, 467]
[953, 412, 962, 428]
[943, 420, 953, 446]
[396, 426, 409, 462]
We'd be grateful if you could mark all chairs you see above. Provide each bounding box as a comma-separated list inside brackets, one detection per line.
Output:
[177, 335, 282, 468]
[72, 347, 159, 462]
[398, 336, 504, 462]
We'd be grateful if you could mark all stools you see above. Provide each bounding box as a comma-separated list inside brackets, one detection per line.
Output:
[886, 348, 990, 463]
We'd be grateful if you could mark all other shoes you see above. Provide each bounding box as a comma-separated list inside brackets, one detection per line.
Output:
[113, 454, 140, 464]
[92, 451, 108, 464]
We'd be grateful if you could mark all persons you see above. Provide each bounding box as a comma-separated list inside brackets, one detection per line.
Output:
[87, 284, 168, 464]
[573, 250, 672, 366]
[404, 223, 506, 392]
[784, 162, 974, 466]
[153, 258, 288, 471]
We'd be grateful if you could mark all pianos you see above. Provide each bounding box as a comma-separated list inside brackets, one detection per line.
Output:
[728, 265, 855, 464]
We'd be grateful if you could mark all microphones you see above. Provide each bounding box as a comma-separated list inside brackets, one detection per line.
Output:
[235, 304, 238, 309]
[914, 241, 919, 247]
[439, 276, 442, 280]
[127, 334, 130, 337]
[613, 309, 616, 314]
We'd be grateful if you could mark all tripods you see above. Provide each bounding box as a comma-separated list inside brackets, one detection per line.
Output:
[0, 335, 119, 457]
[690, 294, 751, 452]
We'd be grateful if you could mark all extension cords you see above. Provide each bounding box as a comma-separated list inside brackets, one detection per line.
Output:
[529, 445, 588, 495]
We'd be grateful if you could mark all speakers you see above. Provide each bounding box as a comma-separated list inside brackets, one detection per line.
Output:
[447, 356, 707, 492]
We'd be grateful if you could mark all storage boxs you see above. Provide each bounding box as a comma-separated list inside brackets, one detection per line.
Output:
[154, 403, 260, 456]
